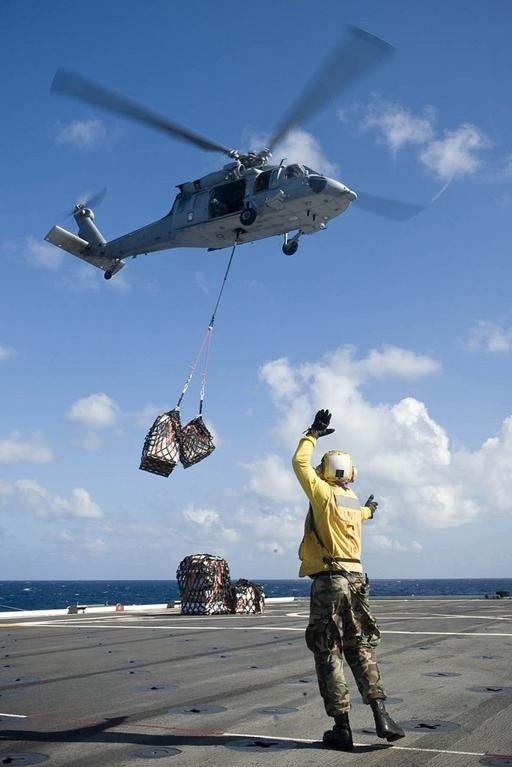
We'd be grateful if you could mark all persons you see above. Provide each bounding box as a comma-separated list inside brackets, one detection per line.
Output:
[289, 409, 407, 752]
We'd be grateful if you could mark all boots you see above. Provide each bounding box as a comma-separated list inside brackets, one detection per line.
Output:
[371, 698, 406, 742]
[323, 712, 354, 751]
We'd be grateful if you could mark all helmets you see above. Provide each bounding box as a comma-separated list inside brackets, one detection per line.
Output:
[320, 449, 356, 484]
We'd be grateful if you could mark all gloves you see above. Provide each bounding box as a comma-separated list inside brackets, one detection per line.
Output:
[307, 409, 335, 439]
[365, 495, 378, 520]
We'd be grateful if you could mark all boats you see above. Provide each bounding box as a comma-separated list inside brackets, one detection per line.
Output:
[0, 589, 512, 767]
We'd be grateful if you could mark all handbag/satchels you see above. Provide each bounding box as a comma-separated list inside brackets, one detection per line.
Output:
[356, 579, 381, 646]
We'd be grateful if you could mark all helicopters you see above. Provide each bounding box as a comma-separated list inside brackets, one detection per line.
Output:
[42, 25, 429, 280]
